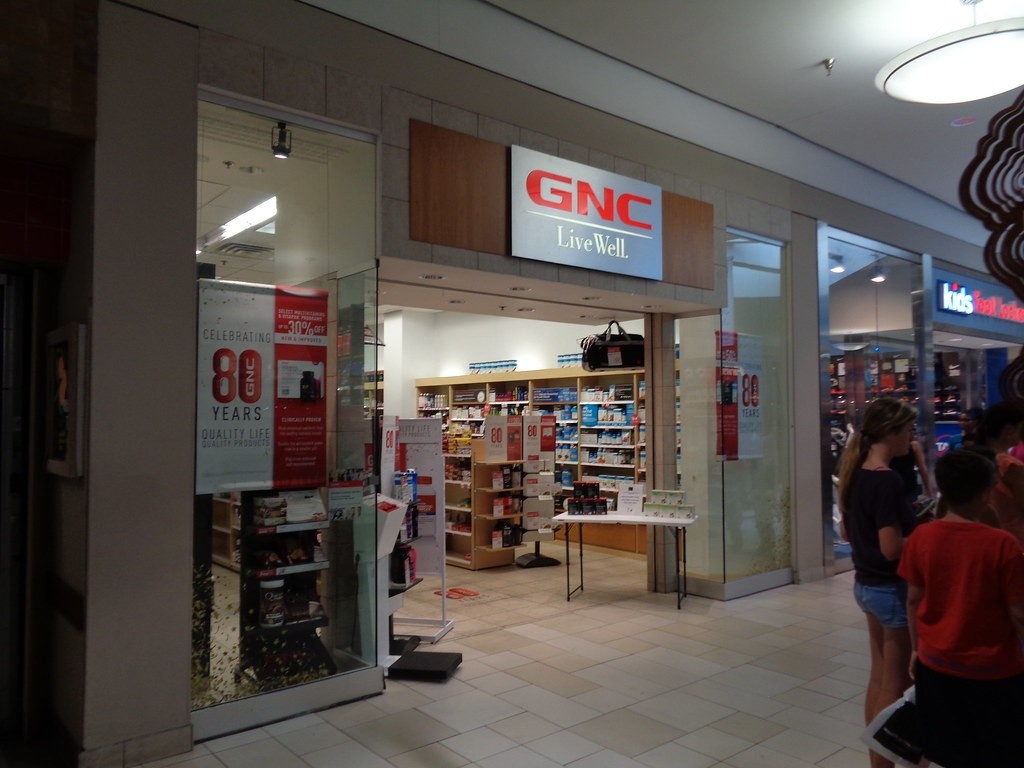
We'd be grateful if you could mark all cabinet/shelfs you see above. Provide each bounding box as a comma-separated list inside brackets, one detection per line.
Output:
[234, 491, 338, 691]
[334, 330, 964, 571]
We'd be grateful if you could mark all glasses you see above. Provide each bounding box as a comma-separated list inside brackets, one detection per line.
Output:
[959, 417, 974, 422]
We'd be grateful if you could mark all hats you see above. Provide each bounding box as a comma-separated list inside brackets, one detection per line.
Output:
[933, 441, 998, 499]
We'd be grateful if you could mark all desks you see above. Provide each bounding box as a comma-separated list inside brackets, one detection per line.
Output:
[549, 508, 699, 609]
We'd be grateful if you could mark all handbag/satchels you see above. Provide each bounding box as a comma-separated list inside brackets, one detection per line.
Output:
[581, 319, 646, 372]
[862, 683, 929, 768]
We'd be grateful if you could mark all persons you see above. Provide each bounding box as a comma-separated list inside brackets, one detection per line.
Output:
[949, 398, 1024, 536]
[897, 449, 1023, 767]
[840, 397, 925, 768]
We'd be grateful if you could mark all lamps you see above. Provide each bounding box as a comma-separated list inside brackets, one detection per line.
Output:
[268, 121, 294, 162]
[829, 253, 846, 276]
[867, 259, 885, 285]
